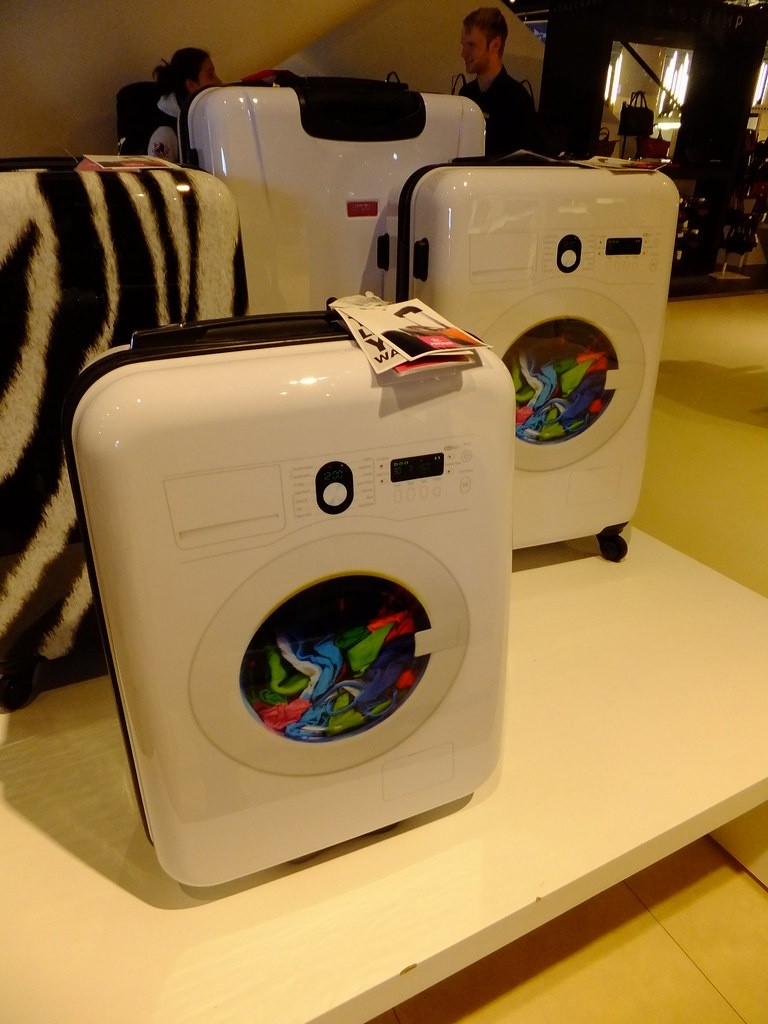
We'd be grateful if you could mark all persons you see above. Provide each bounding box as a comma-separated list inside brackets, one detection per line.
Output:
[141, 47, 223, 162]
[457, 8, 535, 156]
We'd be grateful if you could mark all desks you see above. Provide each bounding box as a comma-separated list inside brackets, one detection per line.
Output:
[1, 528, 768, 1024]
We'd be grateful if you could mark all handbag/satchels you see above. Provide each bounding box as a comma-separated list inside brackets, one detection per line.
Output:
[522, 78, 767, 276]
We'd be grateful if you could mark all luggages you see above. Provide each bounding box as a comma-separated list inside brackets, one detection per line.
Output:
[0, 158, 246, 712]
[176, 64, 488, 312]
[61, 301, 516, 889]
[380, 152, 680, 562]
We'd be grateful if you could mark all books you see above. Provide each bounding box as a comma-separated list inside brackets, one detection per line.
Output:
[334, 298, 492, 377]
